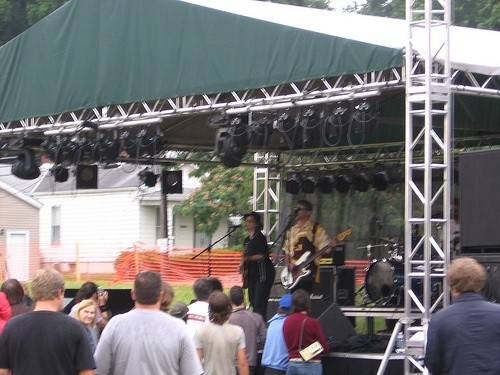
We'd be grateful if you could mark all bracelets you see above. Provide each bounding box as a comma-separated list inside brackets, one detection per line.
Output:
[99, 305, 109, 312]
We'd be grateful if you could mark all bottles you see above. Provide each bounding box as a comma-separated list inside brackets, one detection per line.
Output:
[196, 340, 205, 365]
[396, 331, 405, 349]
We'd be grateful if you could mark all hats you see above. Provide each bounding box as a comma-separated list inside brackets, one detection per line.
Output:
[279, 294, 292, 311]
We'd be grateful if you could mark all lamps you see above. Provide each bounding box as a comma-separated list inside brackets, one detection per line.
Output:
[9, 147, 41, 180]
[140, 167, 159, 187]
[286, 161, 389, 194]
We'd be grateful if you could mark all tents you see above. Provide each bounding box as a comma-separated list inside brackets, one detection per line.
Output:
[0, 0, 500, 164]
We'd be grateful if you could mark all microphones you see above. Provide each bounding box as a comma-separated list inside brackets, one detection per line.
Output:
[230, 224, 242, 229]
[294, 207, 301, 215]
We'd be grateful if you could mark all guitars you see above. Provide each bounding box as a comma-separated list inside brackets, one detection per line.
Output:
[241, 243, 250, 289]
[280, 229, 353, 289]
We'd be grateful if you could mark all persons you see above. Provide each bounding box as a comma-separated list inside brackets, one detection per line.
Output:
[68, 282, 112, 331]
[193, 290, 250, 375]
[282, 199, 330, 302]
[0, 278, 33, 338]
[260, 293, 292, 375]
[425, 257, 500, 375]
[227, 285, 267, 375]
[161, 277, 223, 347]
[93, 271, 206, 375]
[72, 300, 97, 353]
[0, 268, 98, 375]
[238, 212, 276, 323]
[283, 290, 329, 375]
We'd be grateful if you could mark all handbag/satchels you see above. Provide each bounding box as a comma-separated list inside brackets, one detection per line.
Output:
[299, 341, 324, 363]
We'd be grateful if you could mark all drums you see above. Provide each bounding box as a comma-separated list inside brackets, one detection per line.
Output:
[364, 259, 403, 306]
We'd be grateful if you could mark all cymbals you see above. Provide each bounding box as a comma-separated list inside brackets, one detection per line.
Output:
[379, 237, 395, 244]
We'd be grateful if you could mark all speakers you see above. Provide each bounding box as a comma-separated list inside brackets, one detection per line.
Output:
[458, 254, 500, 304]
[161, 170, 183, 195]
[308, 298, 356, 348]
[77, 164, 97, 190]
[458, 149, 499, 251]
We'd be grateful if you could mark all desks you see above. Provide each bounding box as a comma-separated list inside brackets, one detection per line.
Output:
[340, 307, 424, 336]
